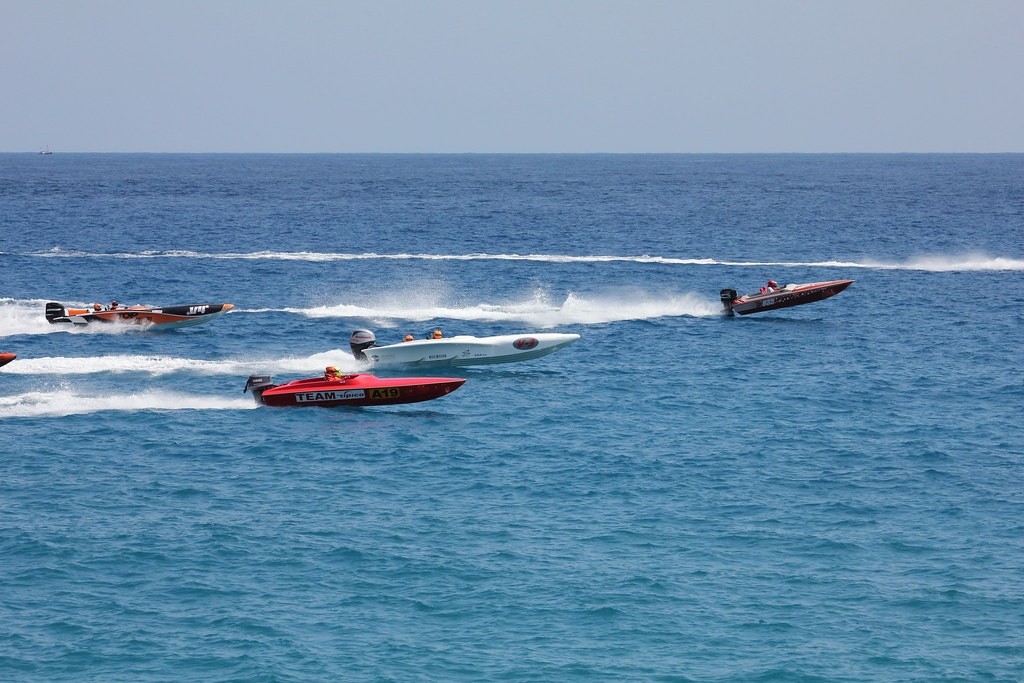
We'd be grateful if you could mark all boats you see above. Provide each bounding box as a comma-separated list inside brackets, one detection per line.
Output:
[719, 279, 856, 317]
[45, 299, 235, 329]
[0, 351, 17, 368]
[242, 365, 467, 406]
[349, 328, 582, 369]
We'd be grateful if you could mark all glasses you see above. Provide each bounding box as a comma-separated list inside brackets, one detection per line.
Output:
[436, 334, 441, 336]
[330, 371, 336, 373]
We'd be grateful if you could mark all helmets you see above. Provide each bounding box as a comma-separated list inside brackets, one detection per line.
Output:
[112, 301, 118, 306]
[326, 367, 336, 371]
[768, 280, 778, 287]
[406, 335, 413, 339]
[94, 304, 102, 310]
[434, 330, 441, 334]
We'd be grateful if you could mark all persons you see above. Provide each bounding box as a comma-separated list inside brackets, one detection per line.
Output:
[430, 329, 443, 339]
[767, 279, 778, 294]
[110, 301, 119, 310]
[94, 302, 102, 312]
[404, 334, 414, 342]
[323, 365, 341, 381]
